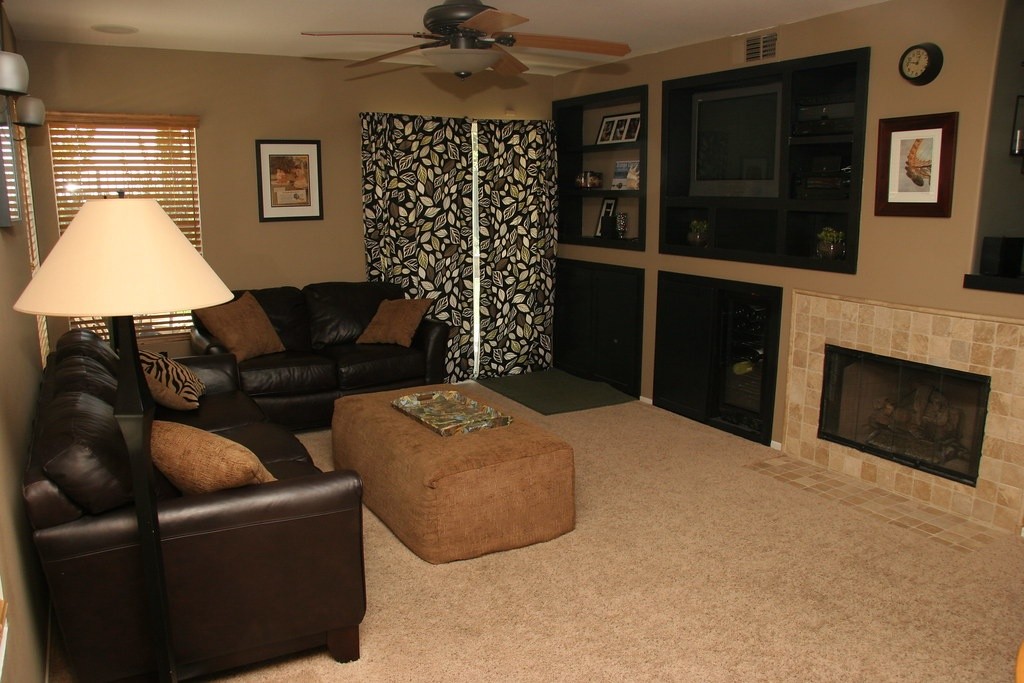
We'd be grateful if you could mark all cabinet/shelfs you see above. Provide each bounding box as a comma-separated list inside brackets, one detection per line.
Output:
[552, 85, 648, 253]
[552, 257, 644, 401]
[654, 272, 783, 448]
[780, 47, 870, 274]
[658, 60, 780, 266]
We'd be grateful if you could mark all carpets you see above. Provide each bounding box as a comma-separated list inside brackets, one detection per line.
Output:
[475, 367, 637, 417]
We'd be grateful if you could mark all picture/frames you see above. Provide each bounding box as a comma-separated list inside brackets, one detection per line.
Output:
[594, 112, 640, 145]
[593, 197, 616, 237]
[253, 140, 323, 222]
[874, 111, 959, 219]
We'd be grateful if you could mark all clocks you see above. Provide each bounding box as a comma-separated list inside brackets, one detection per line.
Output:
[898, 43, 944, 86]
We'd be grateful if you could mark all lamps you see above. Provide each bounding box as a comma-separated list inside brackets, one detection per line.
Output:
[8, 93, 46, 143]
[418, 35, 502, 79]
[0, 50, 28, 95]
[13, 191, 235, 683]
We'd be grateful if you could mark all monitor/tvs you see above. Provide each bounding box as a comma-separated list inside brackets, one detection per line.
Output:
[689, 81, 781, 197]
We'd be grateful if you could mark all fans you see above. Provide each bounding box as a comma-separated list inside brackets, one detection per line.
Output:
[300, 0, 631, 80]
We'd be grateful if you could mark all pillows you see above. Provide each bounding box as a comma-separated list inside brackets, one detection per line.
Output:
[149, 421, 278, 495]
[135, 349, 207, 410]
[191, 293, 286, 362]
[356, 298, 430, 346]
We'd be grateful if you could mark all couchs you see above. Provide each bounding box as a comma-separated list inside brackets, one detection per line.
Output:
[19, 330, 366, 683]
[189, 279, 447, 436]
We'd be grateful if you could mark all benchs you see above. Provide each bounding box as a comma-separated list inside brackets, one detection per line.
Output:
[333, 381, 577, 563]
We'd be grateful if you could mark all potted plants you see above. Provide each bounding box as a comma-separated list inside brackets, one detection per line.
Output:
[817, 227, 845, 261]
[686, 222, 710, 247]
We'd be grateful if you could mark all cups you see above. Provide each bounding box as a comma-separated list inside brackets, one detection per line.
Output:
[601, 216, 618, 239]
[575, 171, 603, 189]
